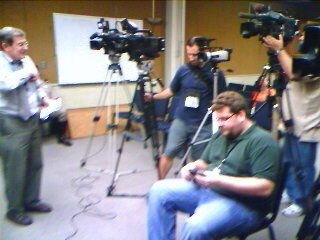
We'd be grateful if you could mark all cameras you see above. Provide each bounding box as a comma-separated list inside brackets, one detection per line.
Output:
[198, 44, 232, 63]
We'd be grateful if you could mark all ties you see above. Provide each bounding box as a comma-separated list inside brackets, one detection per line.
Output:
[12, 60, 32, 121]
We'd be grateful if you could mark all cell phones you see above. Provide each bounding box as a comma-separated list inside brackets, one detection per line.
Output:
[189, 169, 205, 177]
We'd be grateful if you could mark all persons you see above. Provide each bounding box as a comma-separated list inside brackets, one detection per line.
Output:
[0, 26, 52, 225]
[251, 75, 276, 101]
[147, 90, 282, 240]
[263, 20, 320, 218]
[41, 66, 72, 146]
[143, 37, 226, 179]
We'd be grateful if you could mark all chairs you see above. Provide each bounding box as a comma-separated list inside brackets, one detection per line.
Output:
[144, 93, 169, 149]
[114, 90, 147, 148]
[226, 157, 292, 240]
[227, 83, 244, 93]
[245, 86, 254, 93]
[250, 102, 272, 130]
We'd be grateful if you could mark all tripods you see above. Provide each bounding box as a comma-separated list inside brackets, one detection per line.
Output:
[247, 50, 287, 136]
[78, 63, 148, 177]
[106, 64, 164, 196]
[172, 68, 219, 178]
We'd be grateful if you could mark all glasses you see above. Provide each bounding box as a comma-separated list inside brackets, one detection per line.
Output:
[215, 113, 236, 121]
[17, 41, 29, 46]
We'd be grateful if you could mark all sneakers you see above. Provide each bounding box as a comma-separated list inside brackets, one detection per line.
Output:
[7, 209, 32, 225]
[26, 200, 51, 212]
[281, 202, 304, 217]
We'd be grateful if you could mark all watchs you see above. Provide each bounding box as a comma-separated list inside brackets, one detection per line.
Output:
[274, 48, 283, 55]
